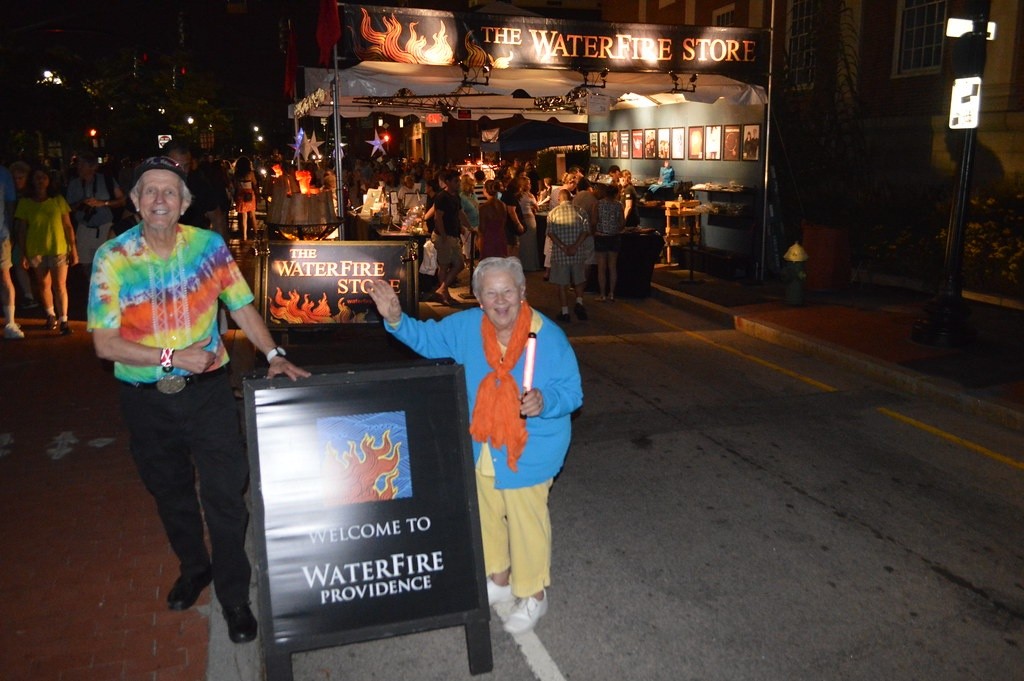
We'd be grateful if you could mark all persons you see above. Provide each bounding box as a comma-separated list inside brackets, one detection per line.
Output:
[661, 132, 669, 158]
[367, 257, 584, 633]
[160, 140, 335, 346]
[646, 132, 654, 157]
[341, 154, 538, 304]
[743, 128, 758, 159]
[599, 133, 617, 158]
[0, 152, 126, 345]
[87, 156, 311, 643]
[709, 127, 718, 159]
[538, 163, 639, 319]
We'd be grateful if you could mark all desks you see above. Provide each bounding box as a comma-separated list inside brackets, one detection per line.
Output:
[588, 178, 683, 199]
[589, 230, 665, 297]
[687, 188, 759, 205]
[375, 227, 433, 287]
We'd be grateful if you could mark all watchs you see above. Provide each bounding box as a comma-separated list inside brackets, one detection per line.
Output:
[266, 346, 287, 363]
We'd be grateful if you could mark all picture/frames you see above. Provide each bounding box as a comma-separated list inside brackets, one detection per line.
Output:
[631, 129, 644, 159]
[671, 127, 685, 159]
[743, 124, 759, 161]
[589, 132, 599, 158]
[619, 130, 630, 159]
[644, 129, 658, 159]
[688, 126, 703, 159]
[598, 131, 609, 158]
[705, 124, 721, 160]
[657, 128, 670, 159]
[723, 125, 741, 161]
[608, 131, 619, 158]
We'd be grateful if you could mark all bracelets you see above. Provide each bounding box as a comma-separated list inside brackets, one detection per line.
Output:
[387, 321, 400, 326]
[160, 347, 175, 367]
[104, 200, 109, 207]
[468, 225, 474, 230]
[560, 244, 565, 248]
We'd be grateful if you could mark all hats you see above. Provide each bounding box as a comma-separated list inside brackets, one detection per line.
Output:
[131, 156, 188, 188]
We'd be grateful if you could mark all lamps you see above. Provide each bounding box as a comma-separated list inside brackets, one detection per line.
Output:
[456, 61, 490, 85]
[577, 67, 609, 87]
[668, 71, 699, 93]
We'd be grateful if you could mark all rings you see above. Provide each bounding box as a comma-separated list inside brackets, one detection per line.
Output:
[537, 403, 541, 408]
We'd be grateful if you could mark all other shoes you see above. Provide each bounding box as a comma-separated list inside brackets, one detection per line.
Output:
[574, 304, 587, 320]
[594, 295, 607, 302]
[556, 311, 570, 322]
[606, 293, 615, 300]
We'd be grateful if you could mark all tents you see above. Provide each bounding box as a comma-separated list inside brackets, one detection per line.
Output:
[283, 4, 777, 295]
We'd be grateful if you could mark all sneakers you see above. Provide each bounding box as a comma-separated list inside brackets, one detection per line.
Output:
[484, 580, 511, 606]
[502, 587, 549, 634]
[4, 323, 26, 340]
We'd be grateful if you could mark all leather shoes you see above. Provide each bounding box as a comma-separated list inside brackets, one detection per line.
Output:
[223, 604, 257, 643]
[167, 566, 214, 610]
[60, 322, 72, 335]
[46, 315, 58, 329]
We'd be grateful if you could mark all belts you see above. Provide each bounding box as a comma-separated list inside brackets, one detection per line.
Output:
[118, 362, 232, 394]
